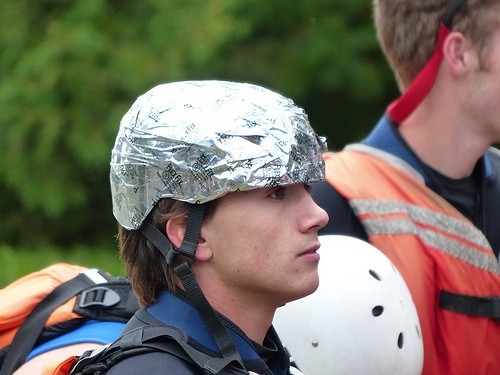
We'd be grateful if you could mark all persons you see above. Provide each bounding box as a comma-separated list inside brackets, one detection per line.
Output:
[306, 0, 500, 374]
[0, 79, 332, 375]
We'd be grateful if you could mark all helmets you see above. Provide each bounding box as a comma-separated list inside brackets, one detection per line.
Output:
[110, 81, 327, 230]
[270, 236, 425, 375]
